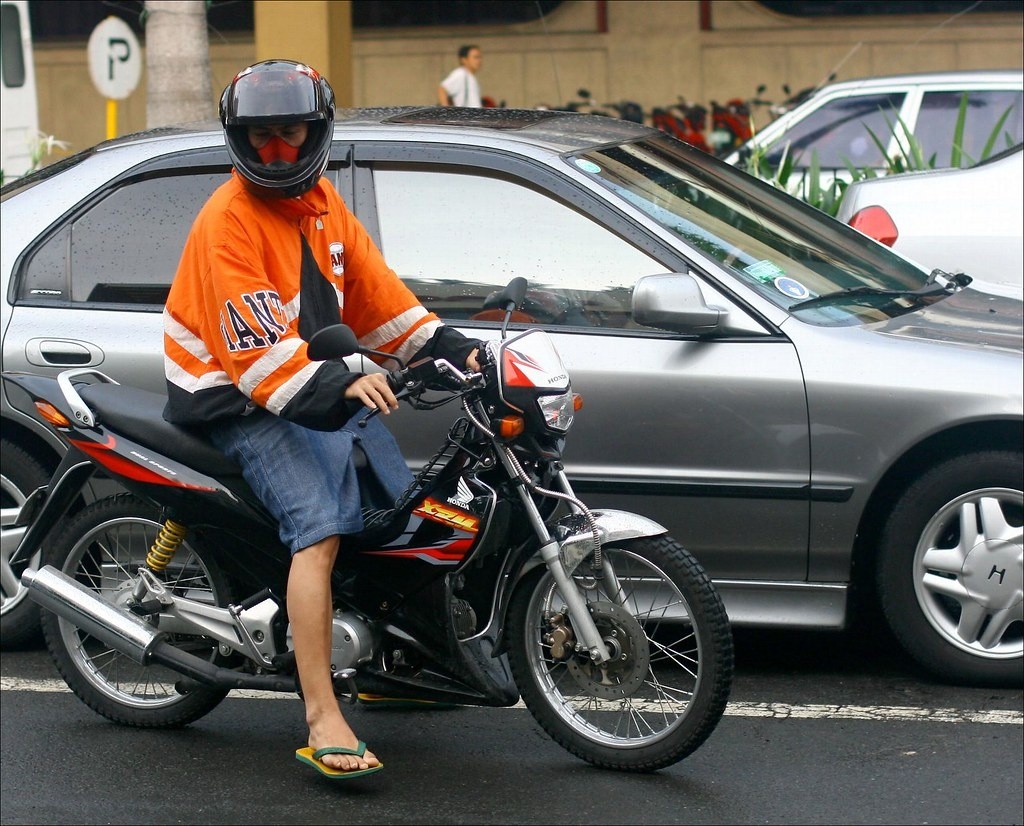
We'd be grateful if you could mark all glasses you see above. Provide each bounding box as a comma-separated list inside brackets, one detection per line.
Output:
[473, 54, 481, 59]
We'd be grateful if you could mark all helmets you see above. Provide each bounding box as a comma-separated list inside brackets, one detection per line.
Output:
[219, 59, 335, 199]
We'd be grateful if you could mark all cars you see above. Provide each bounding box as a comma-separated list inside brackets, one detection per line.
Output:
[718, 70, 1024, 209]
[0, 106, 1023, 689]
[835, 142, 1024, 298]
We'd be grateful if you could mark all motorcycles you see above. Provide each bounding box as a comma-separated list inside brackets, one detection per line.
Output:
[0, 274, 735, 771]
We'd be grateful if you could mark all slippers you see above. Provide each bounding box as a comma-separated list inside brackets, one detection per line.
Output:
[358, 691, 464, 711]
[295, 742, 383, 779]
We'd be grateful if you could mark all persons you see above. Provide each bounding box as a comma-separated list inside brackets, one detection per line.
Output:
[162, 57, 487, 778]
[438, 45, 483, 108]
